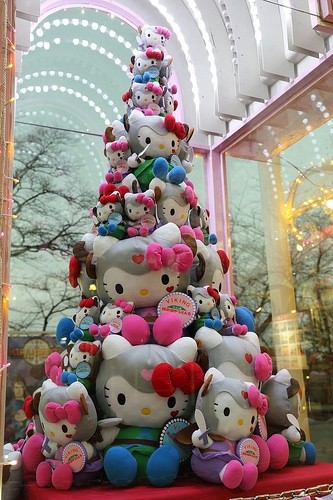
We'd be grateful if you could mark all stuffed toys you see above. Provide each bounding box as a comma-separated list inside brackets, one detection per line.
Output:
[1, 21, 318, 500]
